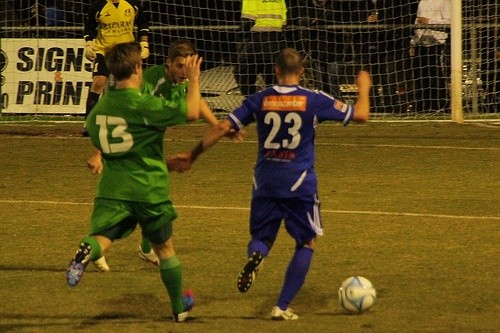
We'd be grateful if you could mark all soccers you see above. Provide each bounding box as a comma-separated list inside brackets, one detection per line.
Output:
[338, 277, 376, 312]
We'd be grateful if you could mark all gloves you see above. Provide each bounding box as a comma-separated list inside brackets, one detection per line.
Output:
[139, 42, 149, 59]
[85, 41, 99, 62]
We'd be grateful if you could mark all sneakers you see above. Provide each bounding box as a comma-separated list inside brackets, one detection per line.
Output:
[236, 250, 265, 293]
[271, 305, 299, 320]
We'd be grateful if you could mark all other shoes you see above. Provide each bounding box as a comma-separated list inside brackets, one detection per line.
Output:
[66, 240, 93, 287]
[171, 288, 196, 323]
[82, 125, 89, 137]
[138, 242, 160, 267]
[94, 255, 109, 271]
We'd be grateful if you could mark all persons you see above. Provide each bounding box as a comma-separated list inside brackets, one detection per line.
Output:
[64, 42, 202, 323]
[167, 48, 371, 320]
[83, 0, 149, 136]
[87, 44, 245, 270]
[0, 0, 500, 113]
